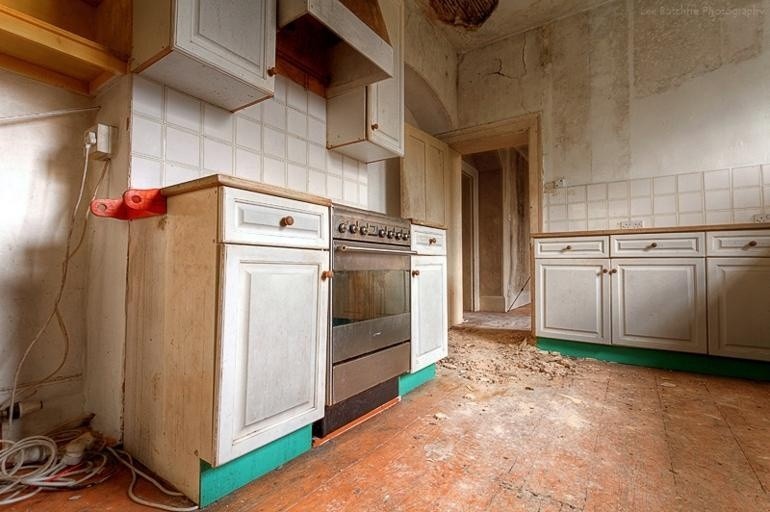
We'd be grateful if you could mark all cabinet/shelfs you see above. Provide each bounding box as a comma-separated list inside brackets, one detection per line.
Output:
[129, 0, 277, 114]
[212, 184, 332, 470]
[530, 230, 707, 357]
[708, 230, 769, 362]
[410, 218, 449, 375]
[326, 1, 406, 164]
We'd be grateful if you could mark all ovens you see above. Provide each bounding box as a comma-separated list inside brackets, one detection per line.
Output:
[330, 214, 418, 366]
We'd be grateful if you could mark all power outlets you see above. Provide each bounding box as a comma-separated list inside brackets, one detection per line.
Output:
[82, 122, 119, 162]
